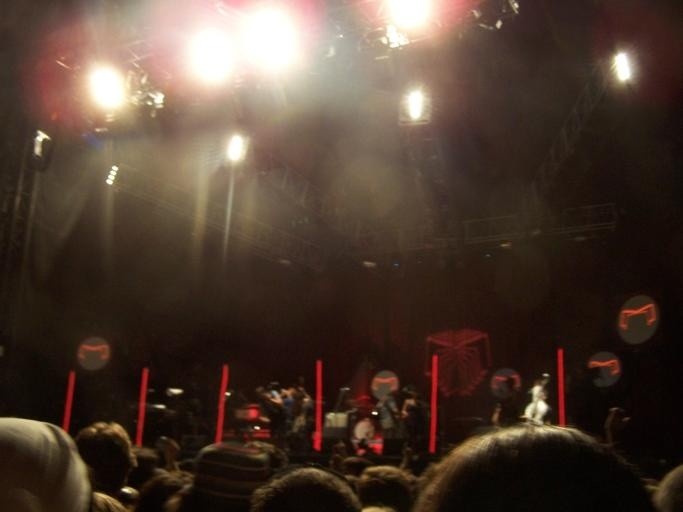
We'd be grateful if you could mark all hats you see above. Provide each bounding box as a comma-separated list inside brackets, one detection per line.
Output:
[185, 442, 272, 499]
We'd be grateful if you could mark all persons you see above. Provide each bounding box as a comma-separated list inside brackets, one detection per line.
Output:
[0, 372, 683, 511]
